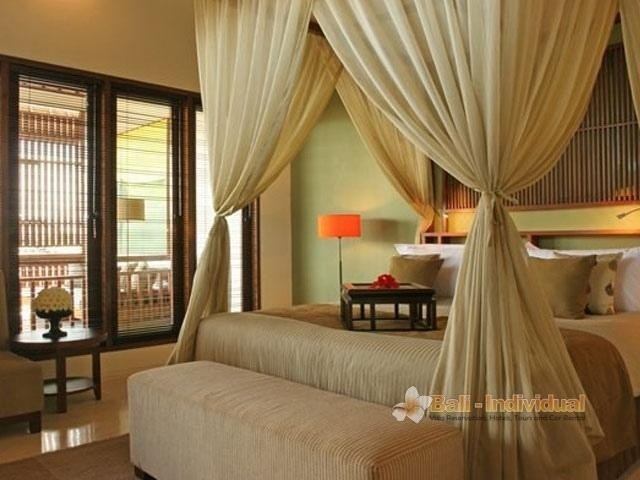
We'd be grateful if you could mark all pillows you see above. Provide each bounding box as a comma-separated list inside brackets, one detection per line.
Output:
[393, 234, 640, 322]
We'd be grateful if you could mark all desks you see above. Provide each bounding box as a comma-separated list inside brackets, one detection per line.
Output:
[339, 280, 438, 332]
[14, 326, 109, 415]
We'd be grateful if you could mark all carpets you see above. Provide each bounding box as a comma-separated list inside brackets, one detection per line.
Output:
[0, 431, 148, 480]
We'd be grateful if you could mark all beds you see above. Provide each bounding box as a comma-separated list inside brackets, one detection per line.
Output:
[186, 245, 639, 480]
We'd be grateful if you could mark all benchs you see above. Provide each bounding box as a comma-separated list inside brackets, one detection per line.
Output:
[123, 358, 468, 479]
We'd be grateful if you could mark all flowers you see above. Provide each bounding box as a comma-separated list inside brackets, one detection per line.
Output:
[30, 285, 73, 313]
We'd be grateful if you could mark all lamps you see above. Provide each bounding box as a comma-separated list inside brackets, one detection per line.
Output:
[116, 198, 145, 251]
[316, 213, 362, 287]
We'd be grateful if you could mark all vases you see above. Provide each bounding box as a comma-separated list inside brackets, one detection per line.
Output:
[37, 310, 73, 339]
